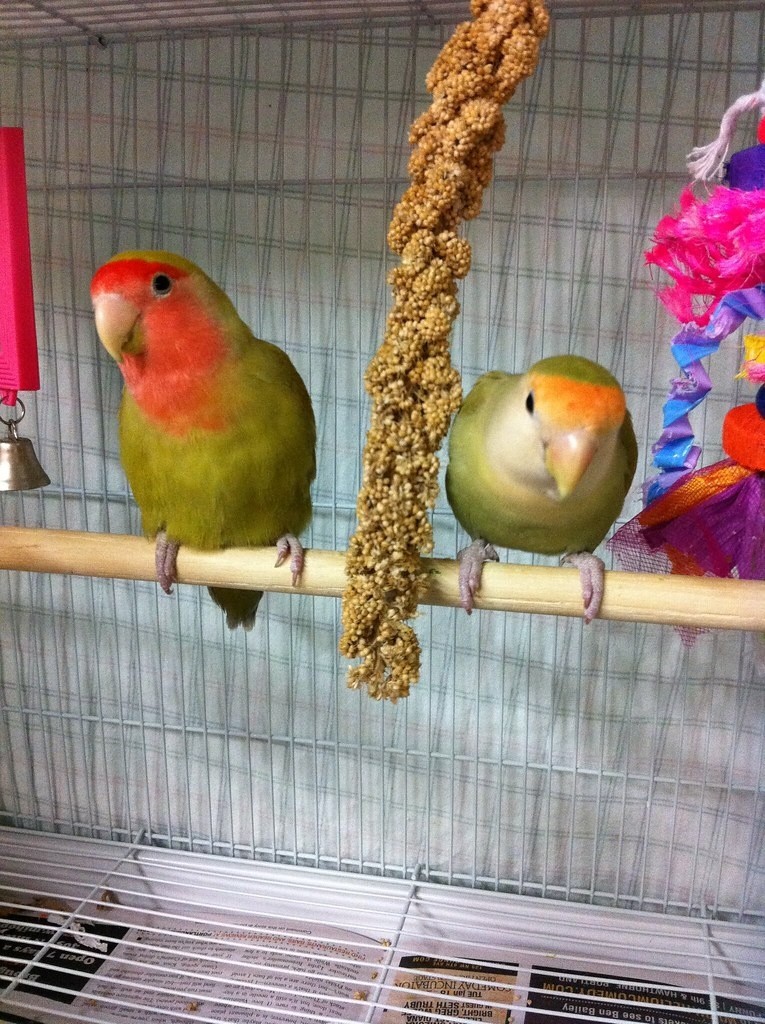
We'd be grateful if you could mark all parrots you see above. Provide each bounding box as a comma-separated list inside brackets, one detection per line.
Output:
[89, 249, 319, 636]
[445, 353, 640, 625]
